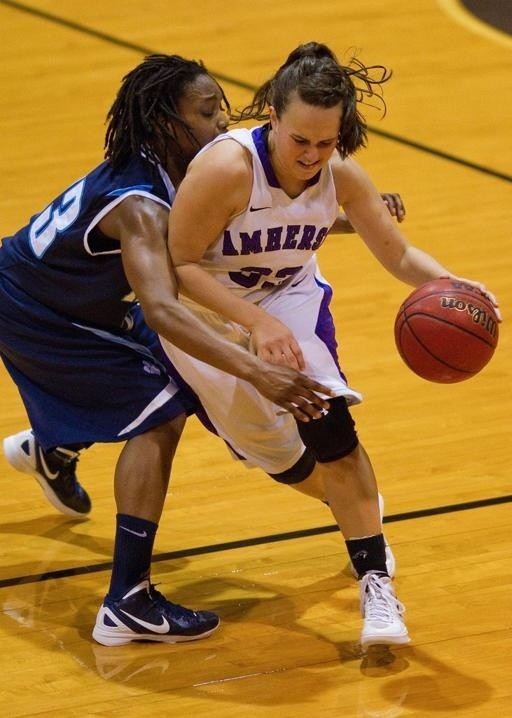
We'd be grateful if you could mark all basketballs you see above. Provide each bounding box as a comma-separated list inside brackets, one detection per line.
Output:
[394, 279, 499, 384]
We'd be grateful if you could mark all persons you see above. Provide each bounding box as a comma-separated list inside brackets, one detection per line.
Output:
[0, 55, 406, 648]
[157, 42, 503, 651]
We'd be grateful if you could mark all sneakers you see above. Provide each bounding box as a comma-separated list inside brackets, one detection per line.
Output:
[4, 427, 93, 518]
[348, 492, 394, 579]
[359, 574, 410, 649]
[92, 579, 221, 647]
